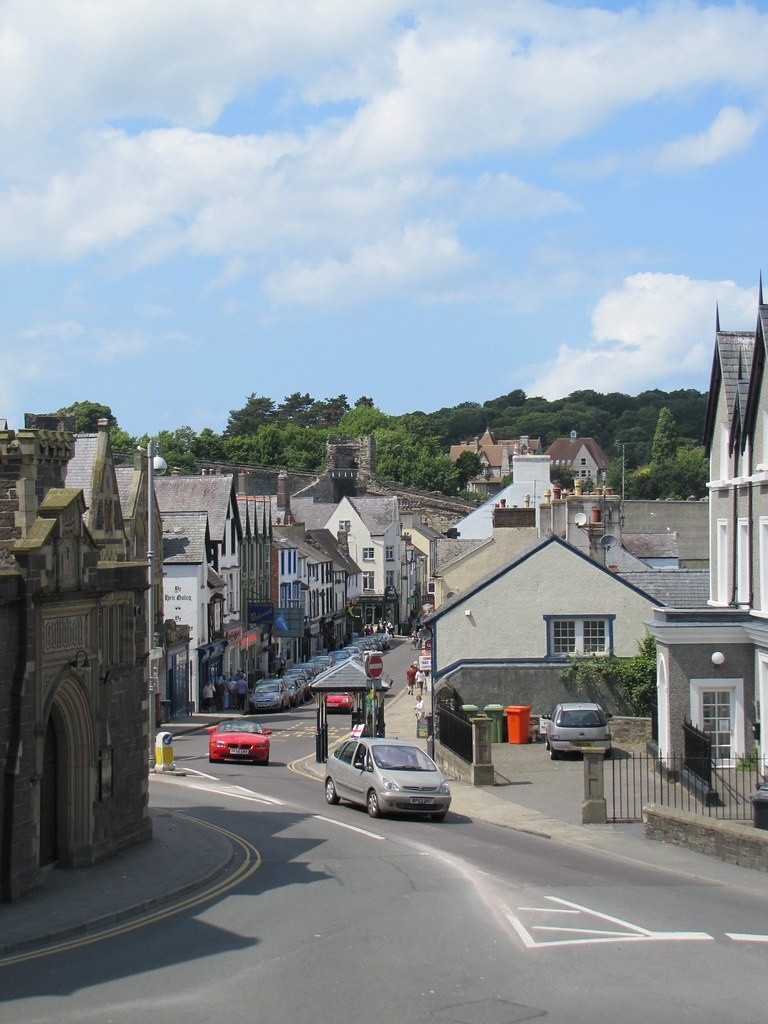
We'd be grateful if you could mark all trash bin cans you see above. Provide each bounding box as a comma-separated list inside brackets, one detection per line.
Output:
[504, 706, 533, 744]
[155, 692, 161, 727]
[352, 711, 363, 730]
[484, 704, 505, 743]
[750, 782, 768, 831]
[400, 623, 410, 635]
[459, 704, 479, 720]
[160, 699, 171, 723]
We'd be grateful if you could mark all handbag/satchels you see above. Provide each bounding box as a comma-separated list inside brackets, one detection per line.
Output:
[202, 699, 208, 710]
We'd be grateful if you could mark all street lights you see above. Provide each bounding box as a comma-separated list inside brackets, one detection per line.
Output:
[147, 442, 168, 767]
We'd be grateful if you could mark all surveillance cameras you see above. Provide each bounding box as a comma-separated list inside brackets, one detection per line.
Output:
[154, 457, 168, 475]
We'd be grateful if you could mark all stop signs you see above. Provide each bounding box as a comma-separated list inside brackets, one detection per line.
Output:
[368, 656, 383, 677]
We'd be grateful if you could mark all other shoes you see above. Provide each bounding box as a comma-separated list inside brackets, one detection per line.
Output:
[408, 691, 410, 694]
[411, 693, 413, 695]
[421, 694, 423, 695]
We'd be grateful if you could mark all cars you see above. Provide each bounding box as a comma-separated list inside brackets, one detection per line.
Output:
[248, 678, 290, 713]
[282, 663, 328, 702]
[342, 647, 363, 660]
[326, 692, 354, 715]
[542, 703, 614, 762]
[347, 633, 391, 651]
[328, 650, 351, 665]
[208, 720, 273, 765]
[284, 678, 305, 706]
[310, 656, 335, 670]
[323, 737, 452, 822]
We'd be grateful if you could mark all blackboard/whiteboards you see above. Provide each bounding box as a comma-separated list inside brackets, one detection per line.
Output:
[417, 718, 428, 738]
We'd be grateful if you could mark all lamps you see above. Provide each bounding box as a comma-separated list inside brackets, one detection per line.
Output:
[161, 527, 185, 535]
[69, 650, 92, 672]
[712, 651, 725, 670]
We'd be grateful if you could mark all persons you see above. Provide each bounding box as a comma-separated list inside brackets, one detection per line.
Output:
[224, 723, 233, 732]
[246, 723, 258, 733]
[365, 620, 395, 638]
[412, 628, 423, 649]
[414, 695, 425, 718]
[407, 661, 425, 696]
[203, 669, 248, 713]
